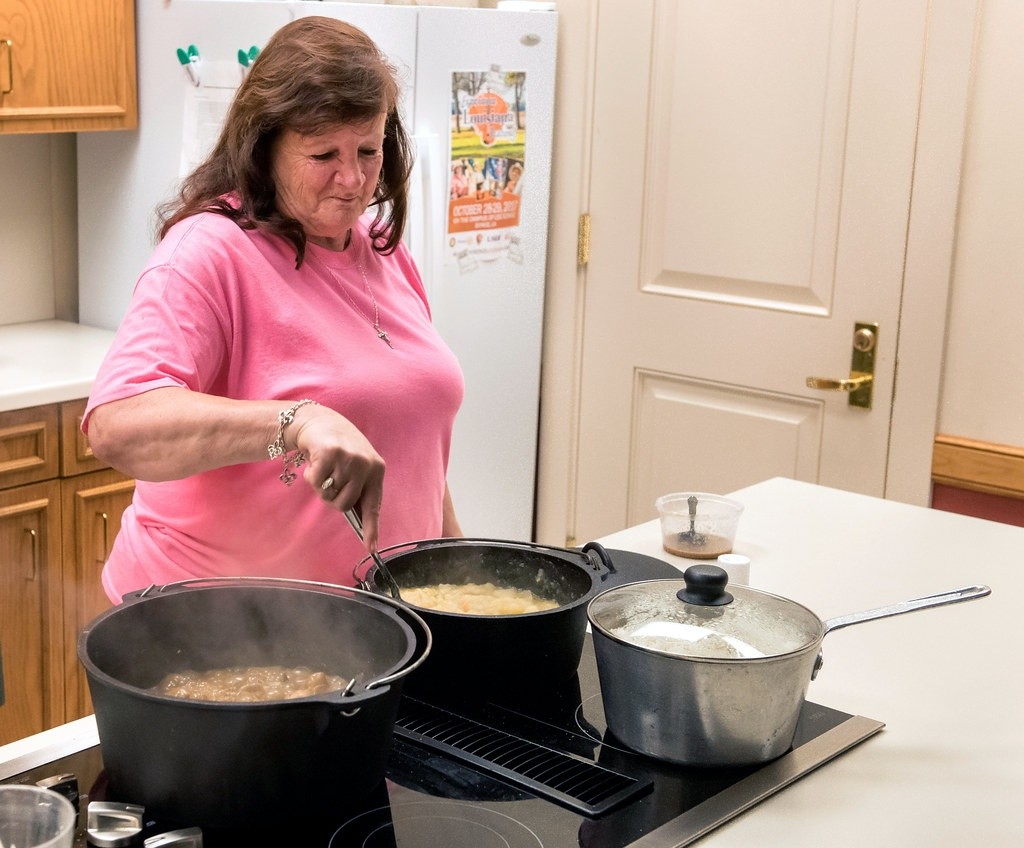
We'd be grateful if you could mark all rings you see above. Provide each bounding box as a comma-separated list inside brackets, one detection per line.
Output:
[322, 477, 341, 495]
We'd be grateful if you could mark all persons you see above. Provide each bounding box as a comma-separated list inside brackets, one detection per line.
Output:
[80, 17, 465, 608]
[505, 162, 522, 192]
[450, 158, 505, 199]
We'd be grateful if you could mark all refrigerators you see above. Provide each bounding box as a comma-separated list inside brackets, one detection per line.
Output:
[80, 0, 562, 542]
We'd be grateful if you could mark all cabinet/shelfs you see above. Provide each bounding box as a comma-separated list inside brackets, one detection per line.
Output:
[0, 401, 199, 747]
[0, 0, 140, 135]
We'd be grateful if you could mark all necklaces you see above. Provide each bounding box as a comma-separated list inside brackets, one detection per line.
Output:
[306, 230, 394, 345]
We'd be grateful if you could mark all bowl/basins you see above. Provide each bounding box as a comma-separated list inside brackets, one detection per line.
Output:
[0, 783, 75, 848]
[656, 491, 745, 559]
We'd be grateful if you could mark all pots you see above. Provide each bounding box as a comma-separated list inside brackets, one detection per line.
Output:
[78, 577, 433, 813]
[589, 565, 991, 763]
[352, 538, 618, 697]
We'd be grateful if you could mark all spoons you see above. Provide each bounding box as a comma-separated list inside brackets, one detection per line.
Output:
[678, 496, 704, 545]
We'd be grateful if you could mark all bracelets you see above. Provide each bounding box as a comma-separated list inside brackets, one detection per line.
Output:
[268, 399, 321, 488]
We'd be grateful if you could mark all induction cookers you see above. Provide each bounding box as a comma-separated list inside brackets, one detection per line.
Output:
[0, 631, 886, 848]
